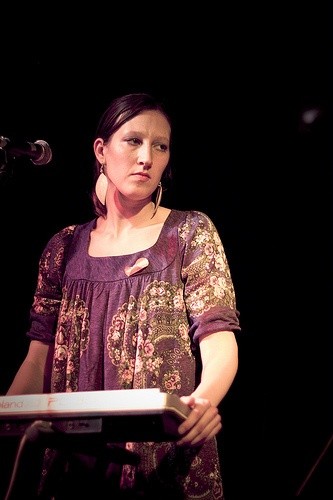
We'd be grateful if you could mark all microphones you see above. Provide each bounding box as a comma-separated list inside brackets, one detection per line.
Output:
[0, 136, 52, 165]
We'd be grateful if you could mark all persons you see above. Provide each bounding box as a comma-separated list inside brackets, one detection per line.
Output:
[0, 94, 242, 500]
